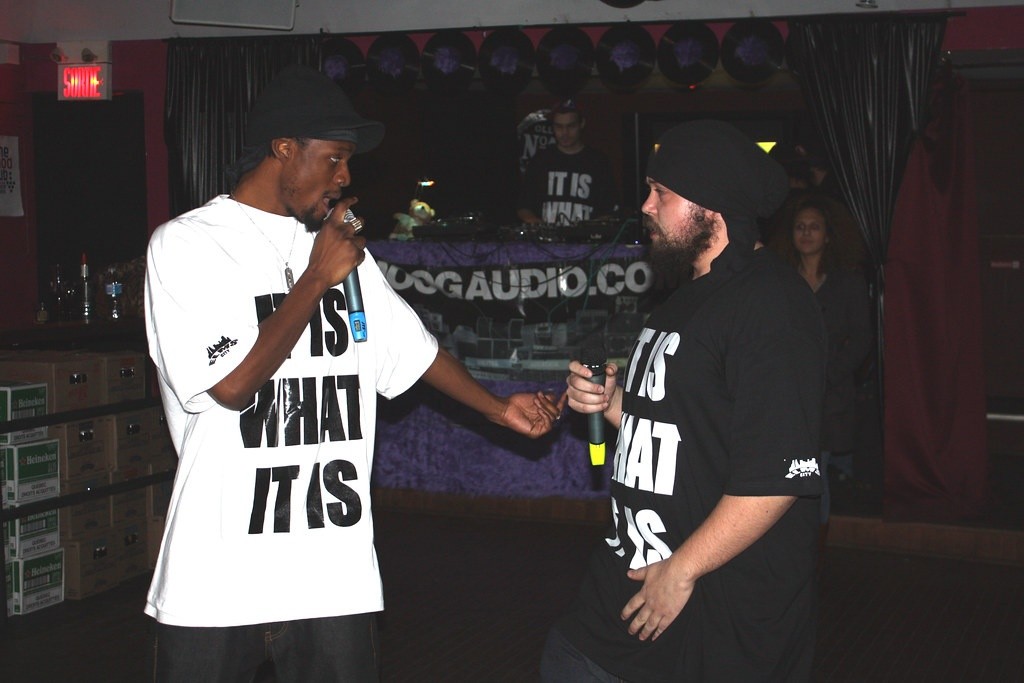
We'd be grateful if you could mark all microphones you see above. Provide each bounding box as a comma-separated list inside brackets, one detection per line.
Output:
[580, 341, 608, 491]
[326, 206, 368, 343]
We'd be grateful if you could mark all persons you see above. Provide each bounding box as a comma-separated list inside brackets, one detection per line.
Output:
[515, 105, 616, 225]
[537, 121, 831, 683]
[145, 58, 562, 683]
[776, 194, 873, 515]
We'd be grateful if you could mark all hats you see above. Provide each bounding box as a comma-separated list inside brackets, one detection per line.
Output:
[245, 63, 386, 155]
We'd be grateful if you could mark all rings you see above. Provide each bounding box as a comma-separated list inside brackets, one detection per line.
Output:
[556, 414, 560, 420]
[349, 217, 362, 235]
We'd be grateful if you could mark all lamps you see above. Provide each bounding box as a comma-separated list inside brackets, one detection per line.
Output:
[413, 177, 436, 202]
[50, 41, 113, 65]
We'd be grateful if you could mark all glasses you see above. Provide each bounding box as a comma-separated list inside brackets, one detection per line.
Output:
[559, 98, 579, 111]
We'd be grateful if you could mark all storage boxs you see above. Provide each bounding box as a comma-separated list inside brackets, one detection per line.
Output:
[23, 350, 146, 405]
[111, 519, 148, 583]
[54, 416, 111, 482]
[0, 381, 48, 446]
[67, 535, 119, 600]
[11, 547, 65, 616]
[150, 458, 178, 520]
[109, 488, 147, 530]
[8, 508, 61, 559]
[67, 496, 110, 541]
[108, 408, 151, 471]
[5, 439, 61, 506]
[149, 517, 165, 569]
[149, 406, 177, 462]
[0, 349, 102, 414]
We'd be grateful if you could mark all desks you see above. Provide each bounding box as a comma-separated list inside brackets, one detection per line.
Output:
[0, 315, 145, 351]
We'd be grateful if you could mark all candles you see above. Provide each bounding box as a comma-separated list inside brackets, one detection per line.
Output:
[80, 253, 89, 277]
